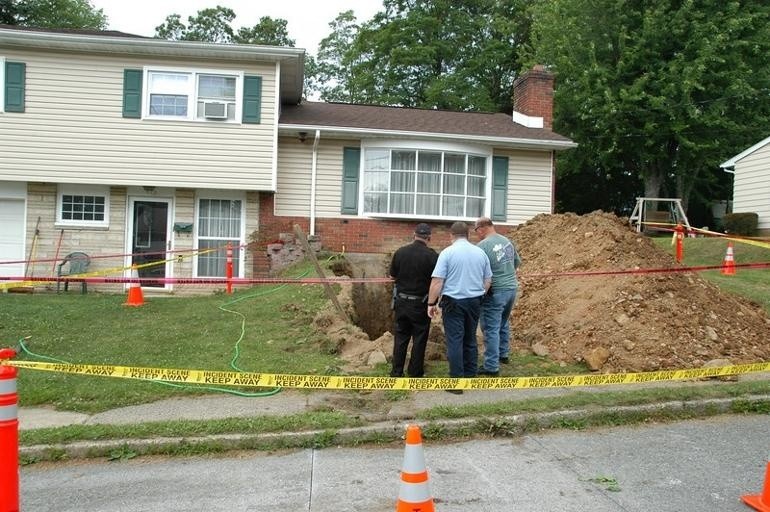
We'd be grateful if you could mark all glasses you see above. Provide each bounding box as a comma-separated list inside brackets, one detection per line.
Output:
[474, 226, 480, 232]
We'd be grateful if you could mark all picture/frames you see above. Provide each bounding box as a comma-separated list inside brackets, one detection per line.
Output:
[134, 202, 153, 249]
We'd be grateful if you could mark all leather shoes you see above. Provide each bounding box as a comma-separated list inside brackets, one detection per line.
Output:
[478, 367, 498, 375]
[500, 357, 508, 362]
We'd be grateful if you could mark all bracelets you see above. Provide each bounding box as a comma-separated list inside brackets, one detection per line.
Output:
[428, 297, 439, 307]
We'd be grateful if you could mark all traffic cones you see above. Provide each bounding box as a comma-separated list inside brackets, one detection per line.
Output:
[739, 459, 770, 512]
[720, 241, 737, 276]
[395, 423, 436, 512]
[121, 262, 152, 307]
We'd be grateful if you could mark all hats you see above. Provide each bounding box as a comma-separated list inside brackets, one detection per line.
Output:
[415, 223, 432, 235]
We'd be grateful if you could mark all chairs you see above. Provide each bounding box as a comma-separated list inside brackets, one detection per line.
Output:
[56, 252, 91, 295]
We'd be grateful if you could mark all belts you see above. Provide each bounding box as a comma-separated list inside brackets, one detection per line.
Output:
[399, 292, 427, 300]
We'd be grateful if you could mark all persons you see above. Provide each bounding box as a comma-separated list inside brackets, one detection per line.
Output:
[388, 222, 439, 377]
[426, 219, 494, 394]
[473, 216, 523, 375]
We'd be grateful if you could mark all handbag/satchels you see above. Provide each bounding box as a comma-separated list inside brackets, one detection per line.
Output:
[438, 296, 455, 318]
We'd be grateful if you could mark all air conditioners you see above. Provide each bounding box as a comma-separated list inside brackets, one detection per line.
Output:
[203, 101, 229, 120]
